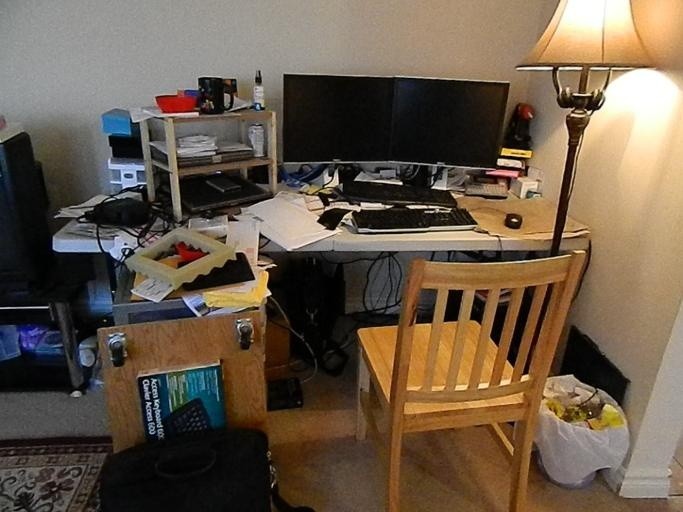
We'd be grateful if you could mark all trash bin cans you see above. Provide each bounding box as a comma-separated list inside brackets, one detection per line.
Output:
[536, 375, 628, 490]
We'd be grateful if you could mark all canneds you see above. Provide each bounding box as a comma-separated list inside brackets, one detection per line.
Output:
[248, 122, 266, 157]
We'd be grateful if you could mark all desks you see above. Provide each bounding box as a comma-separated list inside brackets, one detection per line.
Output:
[53, 184, 589, 377]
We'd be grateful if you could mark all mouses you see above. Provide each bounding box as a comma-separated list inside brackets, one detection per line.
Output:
[505, 213, 522, 229]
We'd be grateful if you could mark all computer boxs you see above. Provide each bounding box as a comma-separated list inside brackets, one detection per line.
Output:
[280, 262, 345, 337]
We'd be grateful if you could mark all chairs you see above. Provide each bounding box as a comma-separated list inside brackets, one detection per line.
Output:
[355, 250, 587, 512]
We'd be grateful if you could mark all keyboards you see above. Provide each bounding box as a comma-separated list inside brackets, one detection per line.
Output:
[343, 181, 458, 208]
[352, 207, 478, 234]
[160, 398, 212, 451]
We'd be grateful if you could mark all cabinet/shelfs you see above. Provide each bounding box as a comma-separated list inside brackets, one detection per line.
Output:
[139, 110, 277, 225]
[0, 253, 116, 397]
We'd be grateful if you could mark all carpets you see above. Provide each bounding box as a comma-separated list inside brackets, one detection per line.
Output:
[0, 436, 113, 512]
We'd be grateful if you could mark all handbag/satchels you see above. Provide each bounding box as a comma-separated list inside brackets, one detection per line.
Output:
[99, 427, 315, 512]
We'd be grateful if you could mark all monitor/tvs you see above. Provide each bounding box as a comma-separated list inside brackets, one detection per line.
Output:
[393, 77, 510, 186]
[282, 72, 395, 182]
[0, 132, 48, 291]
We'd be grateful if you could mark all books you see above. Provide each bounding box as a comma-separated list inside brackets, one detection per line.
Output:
[471, 145, 533, 186]
[149, 134, 254, 170]
[159, 173, 352, 318]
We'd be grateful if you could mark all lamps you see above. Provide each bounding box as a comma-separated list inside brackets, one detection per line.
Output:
[515, 0, 657, 374]
